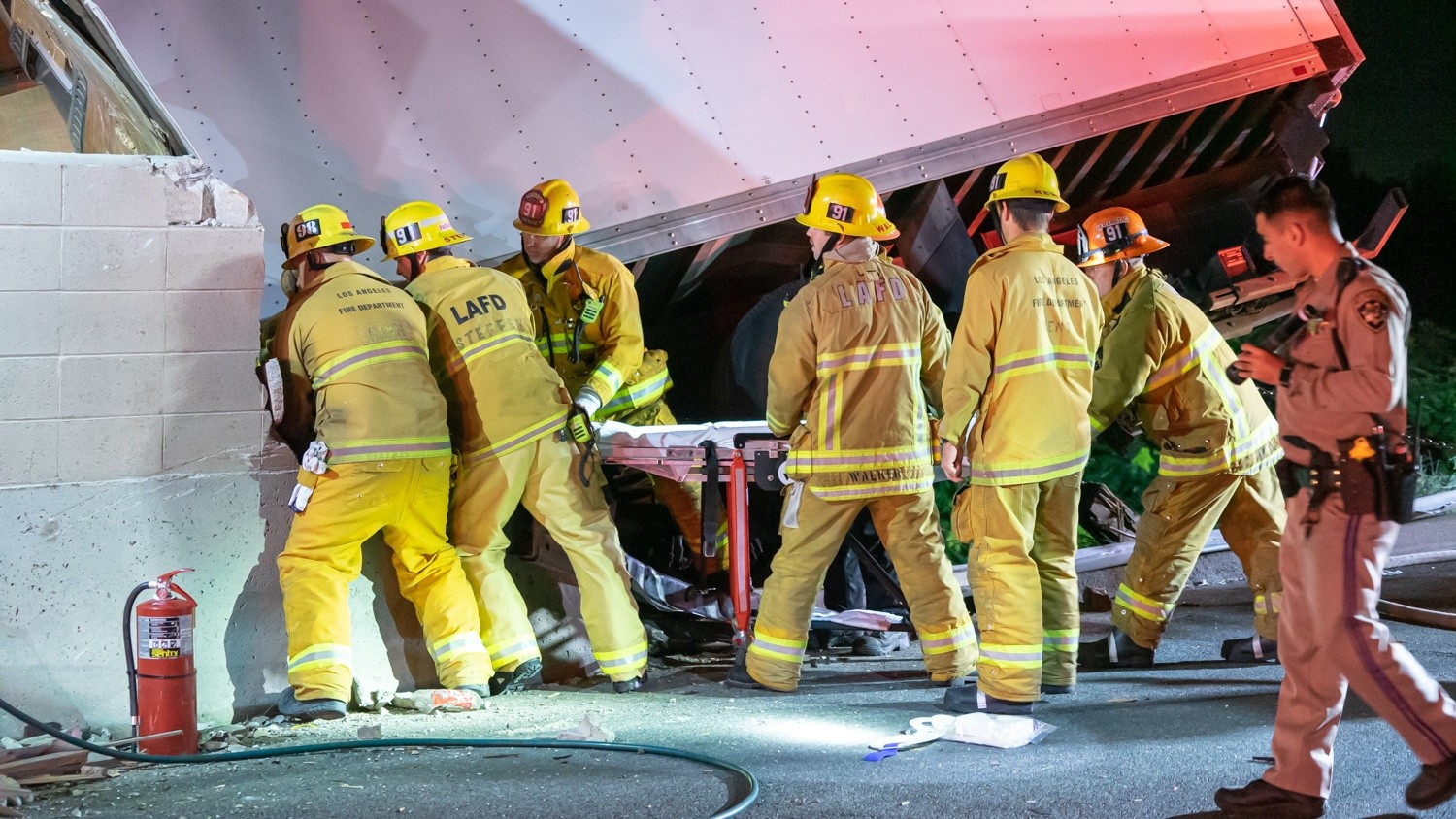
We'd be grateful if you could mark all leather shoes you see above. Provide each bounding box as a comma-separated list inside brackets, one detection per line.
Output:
[932, 669, 979, 686]
[1078, 626, 1155, 671]
[726, 664, 763, 687]
[1221, 635, 1277, 662]
[1042, 685, 1075, 693]
[1215, 779, 1329, 819]
[944, 685, 1033, 714]
[1406, 757, 1456, 810]
[853, 630, 910, 656]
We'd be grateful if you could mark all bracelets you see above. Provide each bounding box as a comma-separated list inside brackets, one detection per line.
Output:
[1279, 358, 1297, 388]
[939, 436, 949, 446]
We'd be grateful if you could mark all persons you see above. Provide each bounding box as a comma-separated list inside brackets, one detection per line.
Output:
[377, 200, 649, 696]
[493, 178, 754, 594]
[1075, 207, 1287, 667]
[936, 152, 1105, 716]
[1213, 174, 1456, 819]
[269, 202, 495, 720]
[726, 172, 981, 692]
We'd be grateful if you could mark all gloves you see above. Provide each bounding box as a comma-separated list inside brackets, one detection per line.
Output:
[287, 441, 329, 513]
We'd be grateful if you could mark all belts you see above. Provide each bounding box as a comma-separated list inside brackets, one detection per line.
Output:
[1284, 457, 1345, 489]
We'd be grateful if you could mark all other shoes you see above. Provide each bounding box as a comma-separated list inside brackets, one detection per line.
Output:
[455, 684, 491, 697]
[488, 657, 540, 695]
[277, 687, 346, 720]
[613, 670, 648, 693]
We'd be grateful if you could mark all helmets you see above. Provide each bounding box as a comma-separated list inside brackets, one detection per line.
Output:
[984, 153, 1070, 213]
[865, 193, 900, 240]
[379, 201, 474, 262]
[513, 179, 591, 236]
[1077, 206, 1171, 270]
[281, 203, 375, 271]
[796, 172, 896, 236]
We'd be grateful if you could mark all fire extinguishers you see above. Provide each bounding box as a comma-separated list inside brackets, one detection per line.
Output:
[122, 567, 197, 756]
